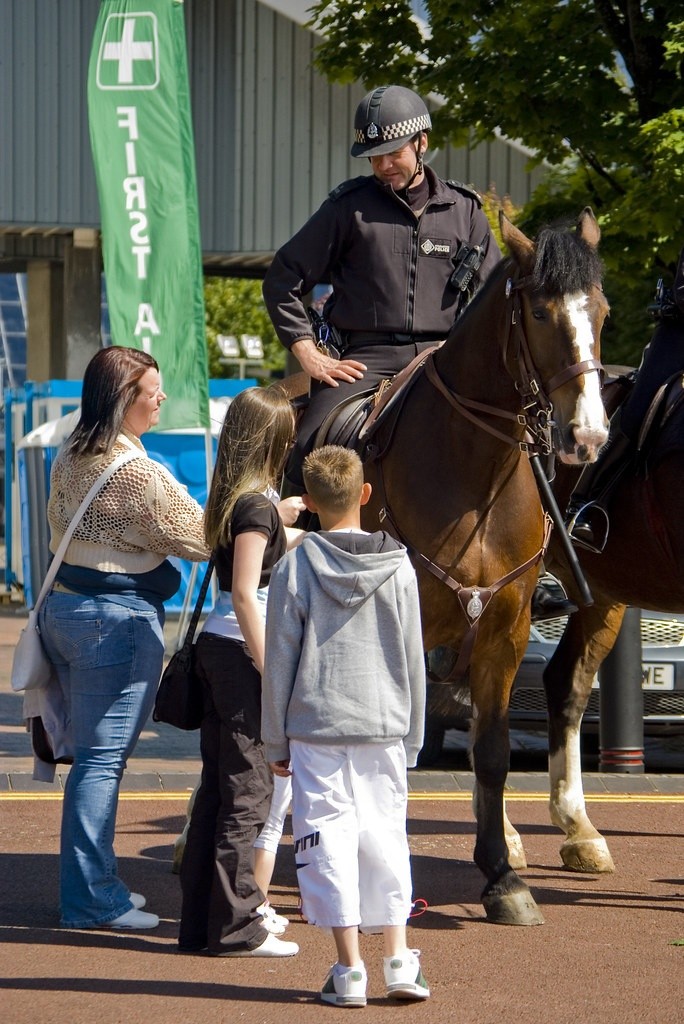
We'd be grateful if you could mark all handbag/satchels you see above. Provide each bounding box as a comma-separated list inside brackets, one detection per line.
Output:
[153, 646, 203, 732]
[9, 609, 50, 692]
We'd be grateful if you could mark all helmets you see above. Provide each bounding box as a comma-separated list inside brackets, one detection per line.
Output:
[350, 84, 433, 159]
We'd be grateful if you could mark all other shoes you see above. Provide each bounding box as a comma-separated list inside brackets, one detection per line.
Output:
[530, 584, 579, 618]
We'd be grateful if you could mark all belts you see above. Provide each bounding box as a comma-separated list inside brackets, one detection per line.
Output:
[345, 330, 436, 346]
[52, 581, 80, 597]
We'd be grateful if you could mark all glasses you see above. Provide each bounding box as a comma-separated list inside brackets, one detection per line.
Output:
[286, 436, 297, 448]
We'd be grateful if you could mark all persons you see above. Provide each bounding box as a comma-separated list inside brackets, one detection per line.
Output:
[177, 386, 308, 957]
[36, 345, 307, 929]
[262, 85, 579, 629]
[260, 454, 430, 1006]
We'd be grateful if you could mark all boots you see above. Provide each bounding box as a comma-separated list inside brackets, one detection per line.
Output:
[559, 404, 636, 546]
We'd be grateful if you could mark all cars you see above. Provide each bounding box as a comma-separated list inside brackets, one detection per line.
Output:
[426, 609, 684, 757]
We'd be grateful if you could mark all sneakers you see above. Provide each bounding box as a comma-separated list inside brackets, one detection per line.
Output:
[199, 931, 299, 958]
[268, 905, 290, 928]
[383, 948, 430, 999]
[127, 891, 147, 910]
[256, 898, 286, 936]
[88, 909, 160, 930]
[320, 961, 369, 1008]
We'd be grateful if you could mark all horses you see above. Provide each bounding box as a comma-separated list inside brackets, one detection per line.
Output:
[172, 201, 684, 925]
